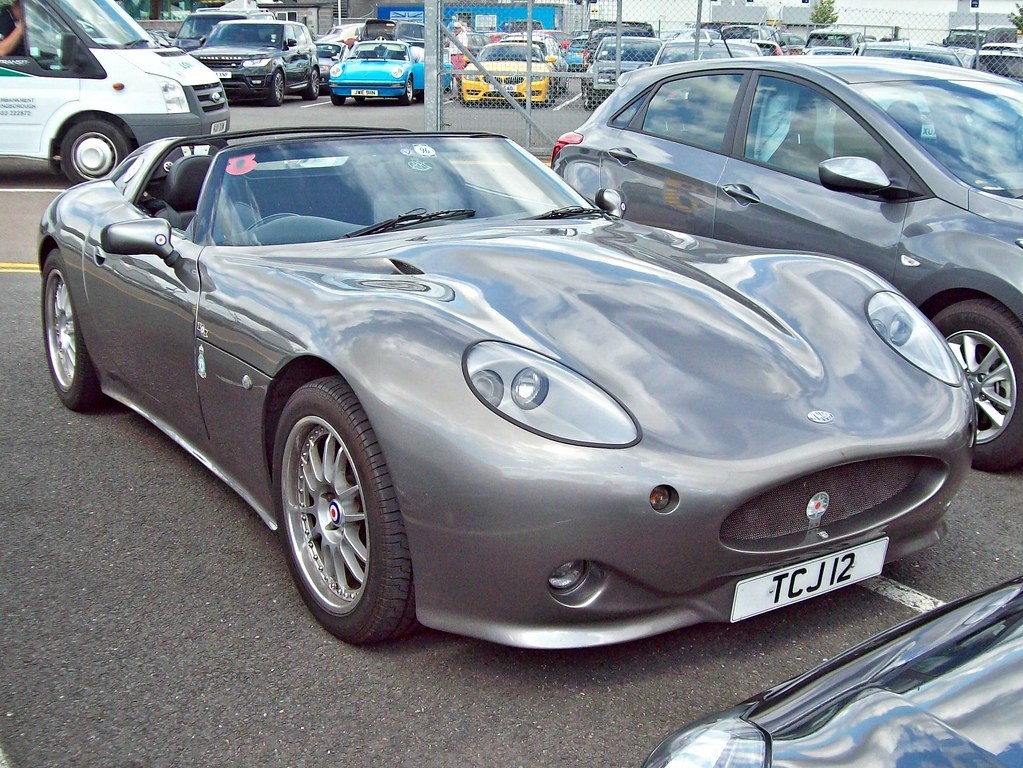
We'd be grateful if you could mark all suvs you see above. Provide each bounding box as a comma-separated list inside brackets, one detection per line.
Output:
[146, 6, 320, 107]
[581, 19, 1022, 110]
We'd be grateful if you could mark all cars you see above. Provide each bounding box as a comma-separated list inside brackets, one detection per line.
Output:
[644, 573, 1023, 768]
[313, 18, 590, 107]
[549, 56, 1023, 473]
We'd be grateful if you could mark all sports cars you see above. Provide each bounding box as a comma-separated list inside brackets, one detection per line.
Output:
[39, 127, 975, 649]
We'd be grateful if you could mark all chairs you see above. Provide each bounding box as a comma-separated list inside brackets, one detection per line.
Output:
[878, 99, 931, 196]
[150, 154, 262, 246]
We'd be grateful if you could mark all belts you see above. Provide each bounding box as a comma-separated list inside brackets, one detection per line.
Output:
[451, 54, 463, 56]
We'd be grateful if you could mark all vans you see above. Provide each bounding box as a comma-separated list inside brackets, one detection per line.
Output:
[0, 0, 230, 186]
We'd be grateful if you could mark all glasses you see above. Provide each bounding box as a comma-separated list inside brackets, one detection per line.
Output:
[453, 27, 460, 29]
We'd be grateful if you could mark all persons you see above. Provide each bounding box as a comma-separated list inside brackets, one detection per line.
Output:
[342, 35, 358, 62]
[444, 16, 473, 101]
[410, 46, 424, 102]
[0, 0, 52, 70]
